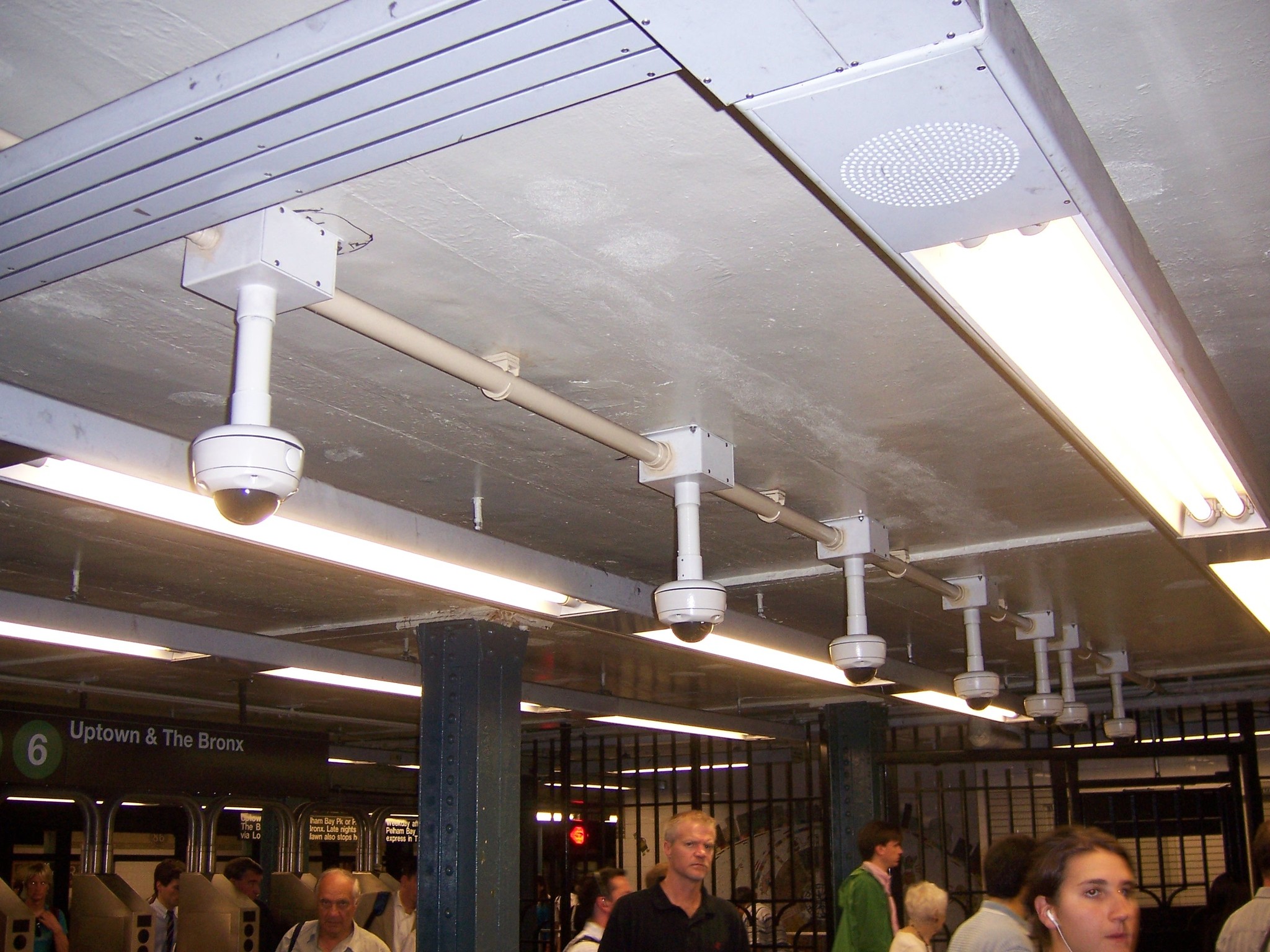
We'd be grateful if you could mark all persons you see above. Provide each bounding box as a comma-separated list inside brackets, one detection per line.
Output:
[537, 811, 1270, 952]
[149, 860, 187, 952]
[275, 867, 392, 952]
[23, 863, 69, 952]
[353, 856, 416, 952]
[224, 857, 272, 952]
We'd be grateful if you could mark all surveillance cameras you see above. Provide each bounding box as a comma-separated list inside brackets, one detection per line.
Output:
[953, 671, 1000, 710]
[653, 579, 727, 645]
[189, 424, 304, 526]
[1023, 693, 1137, 746]
[829, 635, 887, 684]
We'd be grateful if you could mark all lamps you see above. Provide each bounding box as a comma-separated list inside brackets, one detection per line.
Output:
[1, 381, 1040, 724]
[1, 589, 785, 740]
[615, 0, 1270, 636]
